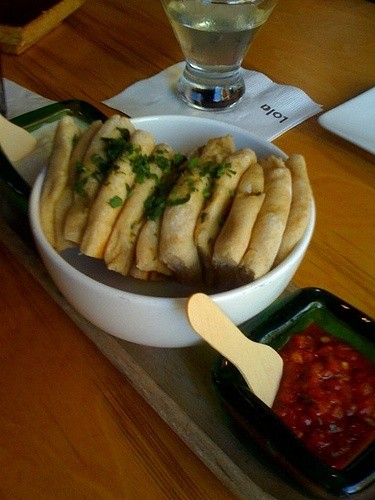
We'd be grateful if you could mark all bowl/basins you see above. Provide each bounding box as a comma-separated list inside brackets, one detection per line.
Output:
[30, 115, 315, 347]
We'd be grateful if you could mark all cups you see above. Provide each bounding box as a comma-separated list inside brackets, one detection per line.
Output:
[162, 0, 278, 112]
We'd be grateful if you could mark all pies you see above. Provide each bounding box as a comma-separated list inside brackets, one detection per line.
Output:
[37, 113, 314, 282]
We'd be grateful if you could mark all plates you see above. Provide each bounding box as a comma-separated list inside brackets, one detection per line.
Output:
[317, 86, 375, 155]
[0, 99, 109, 220]
[210, 287, 375, 496]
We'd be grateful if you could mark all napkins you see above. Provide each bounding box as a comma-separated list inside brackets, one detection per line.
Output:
[101, 63, 324, 143]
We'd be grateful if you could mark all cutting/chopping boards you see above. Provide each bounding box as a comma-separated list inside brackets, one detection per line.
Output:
[0, 0, 375, 500]
